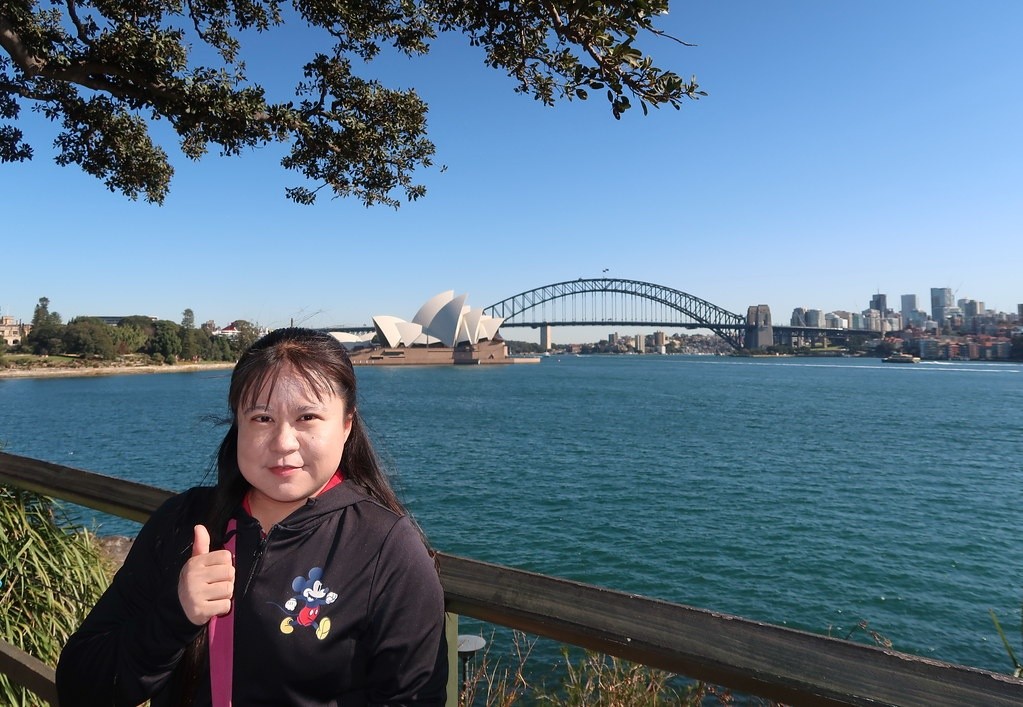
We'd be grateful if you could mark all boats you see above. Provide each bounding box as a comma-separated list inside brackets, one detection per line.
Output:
[881, 350, 921, 365]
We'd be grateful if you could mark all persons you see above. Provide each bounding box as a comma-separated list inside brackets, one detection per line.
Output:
[55, 326, 449, 707]
